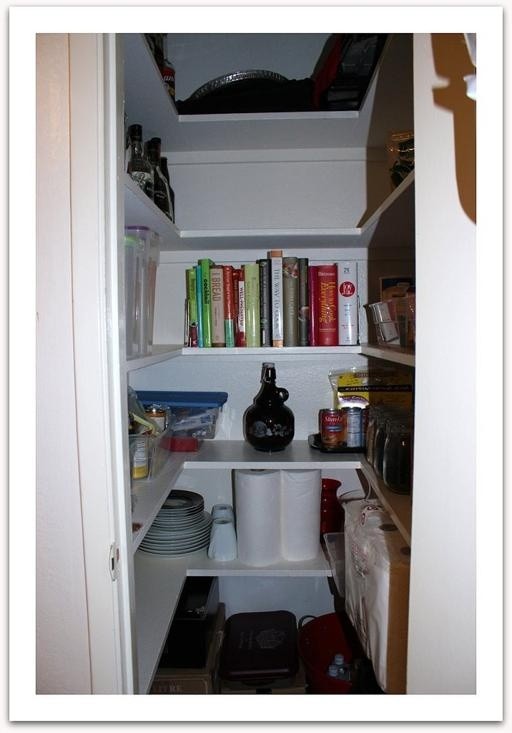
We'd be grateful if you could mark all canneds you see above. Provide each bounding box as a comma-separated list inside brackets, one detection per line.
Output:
[319, 407, 368, 448]
[130, 434, 149, 479]
[144, 404, 171, 431]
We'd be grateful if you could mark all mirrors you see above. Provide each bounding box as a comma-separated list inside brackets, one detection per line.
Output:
[428, 31, 477, 224]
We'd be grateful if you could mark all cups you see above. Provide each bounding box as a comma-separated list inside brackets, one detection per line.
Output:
[212, 502, 235, 521]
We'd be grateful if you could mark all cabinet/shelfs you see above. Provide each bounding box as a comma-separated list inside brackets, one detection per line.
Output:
[362, 32, 477, 696]
[115, 31, 185, 696]
[182, 108, 363, 581]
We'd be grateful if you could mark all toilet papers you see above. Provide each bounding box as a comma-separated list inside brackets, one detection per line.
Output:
[281, 469, 320, 562]
[235, 470, 281, 568]
[213, 503, 231, 519]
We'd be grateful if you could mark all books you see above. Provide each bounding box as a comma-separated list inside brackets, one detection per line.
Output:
[183, 248, 362, 347]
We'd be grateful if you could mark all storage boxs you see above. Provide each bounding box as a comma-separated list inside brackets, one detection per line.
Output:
[221, 659, 306, 694]
[124, 224, 163, 356]
[149, 601, 227, 694]
[122, 235, 145, 360]
[337, 487, 412, 695]
[135, 390, 228, 440]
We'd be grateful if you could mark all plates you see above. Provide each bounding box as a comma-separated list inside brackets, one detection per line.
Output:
[136, 491, 214, 559]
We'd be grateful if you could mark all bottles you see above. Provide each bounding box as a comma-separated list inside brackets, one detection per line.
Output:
[366, 403, 411, 495]
[328, 653, 351, 680]
[242, 362, 294, 454]
[126, 119, 179, 222]
[320, 478, 344, 546]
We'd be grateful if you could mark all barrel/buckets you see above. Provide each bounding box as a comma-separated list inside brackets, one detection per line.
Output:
[298, 612, 375, 693]
